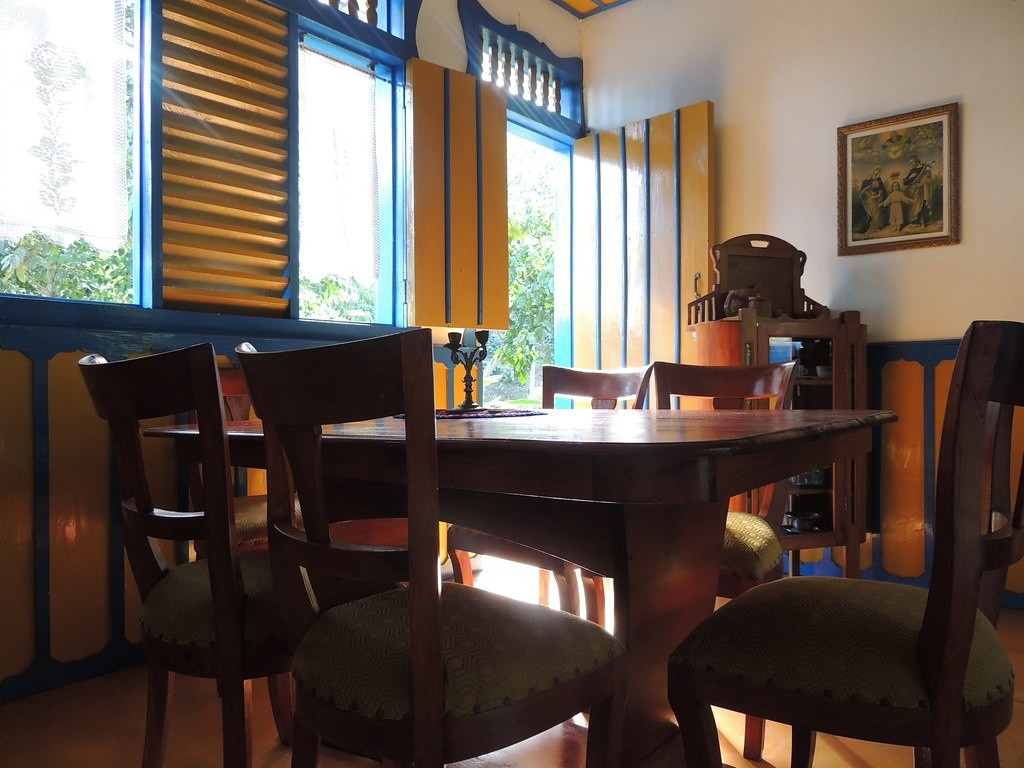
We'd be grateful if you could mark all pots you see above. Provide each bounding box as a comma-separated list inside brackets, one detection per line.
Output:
[785, 510, 824, 530]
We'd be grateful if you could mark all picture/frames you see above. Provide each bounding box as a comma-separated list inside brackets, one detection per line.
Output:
[835, 102, 958, 256]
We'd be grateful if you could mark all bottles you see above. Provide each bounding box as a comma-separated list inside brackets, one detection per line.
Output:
[792, 467, 823, 485]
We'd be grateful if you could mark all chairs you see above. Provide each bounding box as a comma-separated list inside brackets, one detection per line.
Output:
[177, 365, 304, 696]
[74, 338, 313, 768]
[239, 327, 622, 768]
[444, 363, 649, 629]
[663, 311, 1024, 768]
[585, 359, 795, 760]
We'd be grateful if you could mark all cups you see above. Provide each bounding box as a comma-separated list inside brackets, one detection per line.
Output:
[795, 365, 808, 376]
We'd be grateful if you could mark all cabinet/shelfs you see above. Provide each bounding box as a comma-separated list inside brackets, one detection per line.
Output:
[680, 317, 870, 580]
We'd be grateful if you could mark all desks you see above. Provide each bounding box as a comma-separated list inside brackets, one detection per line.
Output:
[138, 410, 896, 768]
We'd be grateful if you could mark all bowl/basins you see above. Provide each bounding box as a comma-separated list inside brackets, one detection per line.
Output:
[816, 365, 833, 378]
